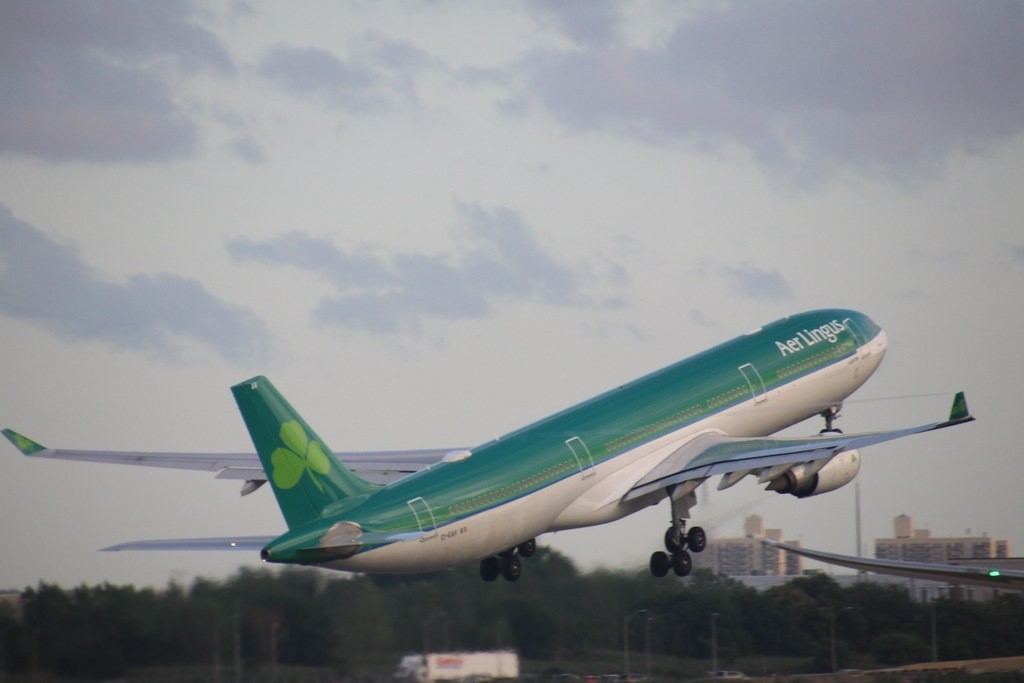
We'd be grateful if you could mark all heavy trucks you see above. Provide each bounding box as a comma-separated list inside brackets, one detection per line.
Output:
[394, 649, 520, 683]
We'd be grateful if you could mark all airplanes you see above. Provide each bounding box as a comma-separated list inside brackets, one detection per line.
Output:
[0, 309, 976, 582]
[757, 536, 1023, 585]
